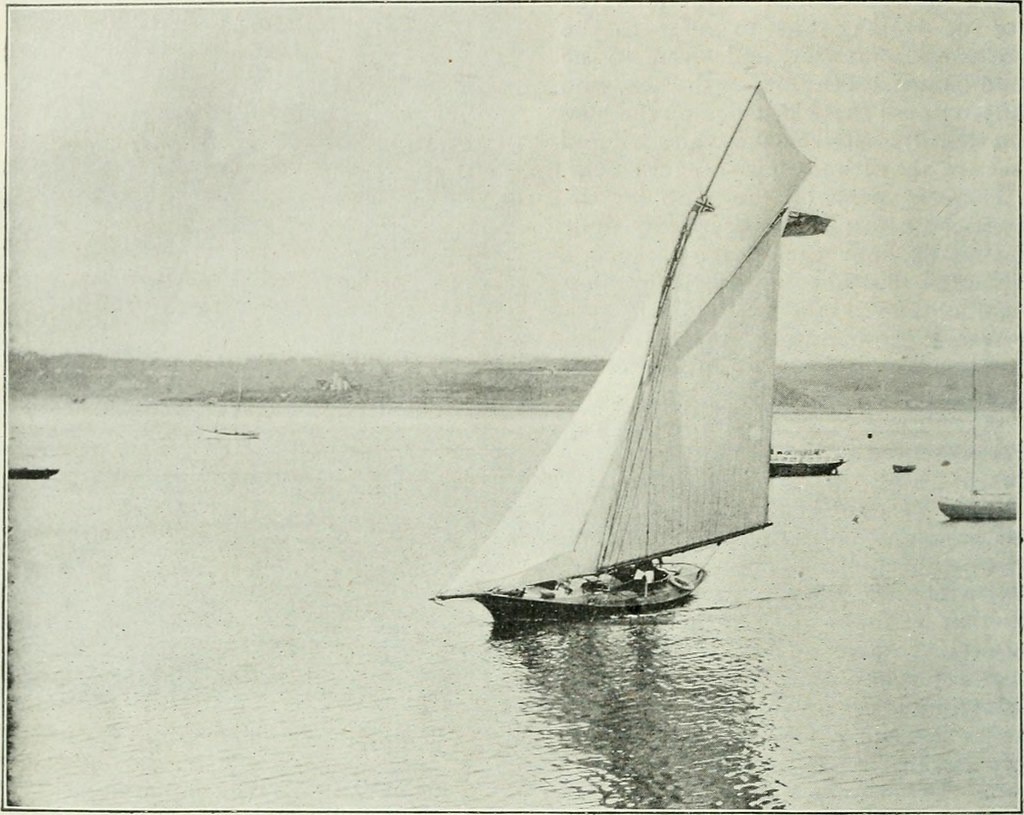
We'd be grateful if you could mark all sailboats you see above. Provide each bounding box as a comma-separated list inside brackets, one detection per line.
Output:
[426, 79, 822, 624]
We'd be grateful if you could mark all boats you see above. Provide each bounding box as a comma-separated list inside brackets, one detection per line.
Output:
[195, 386, 260, 440]
[766, 446, 847, 476]
[9, 467, 57, 478]
[892, 464, 916, 472]
[936, 360, 1020, 519]
